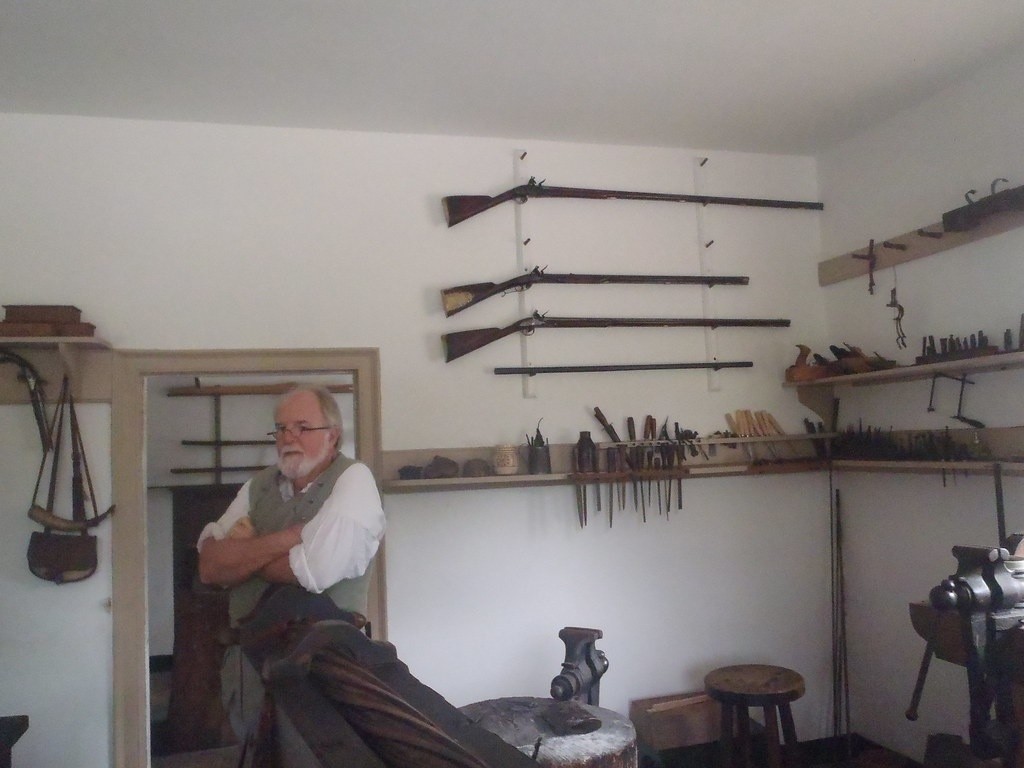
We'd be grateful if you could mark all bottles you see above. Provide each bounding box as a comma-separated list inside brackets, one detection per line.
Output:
[578, 431, 596, 472]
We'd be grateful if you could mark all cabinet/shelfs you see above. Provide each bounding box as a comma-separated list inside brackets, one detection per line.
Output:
[782, 351, 1024, 478]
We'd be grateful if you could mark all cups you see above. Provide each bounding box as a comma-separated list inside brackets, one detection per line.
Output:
[494, 444, 518, 475]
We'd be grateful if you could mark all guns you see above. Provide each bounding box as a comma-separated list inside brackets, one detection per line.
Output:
[442, 269, 755, 316]
[443, 314, 794, 365]
[444, 178, 826, 231]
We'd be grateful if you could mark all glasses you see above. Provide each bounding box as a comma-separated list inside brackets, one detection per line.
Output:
[266, 426, 331, 439]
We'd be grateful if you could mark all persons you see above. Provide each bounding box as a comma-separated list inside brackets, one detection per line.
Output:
[196, 384, 387, 768]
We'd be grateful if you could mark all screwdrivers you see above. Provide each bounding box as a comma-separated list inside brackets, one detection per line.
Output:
[567, 404, 687, 529]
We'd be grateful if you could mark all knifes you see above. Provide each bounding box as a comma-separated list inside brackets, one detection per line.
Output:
[572, 449, 584, 529]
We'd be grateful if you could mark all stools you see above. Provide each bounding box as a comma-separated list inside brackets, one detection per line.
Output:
[704, 664, 807, 768]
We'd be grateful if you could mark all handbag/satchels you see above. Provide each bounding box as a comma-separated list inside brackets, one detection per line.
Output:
[27, 532, 98, 585]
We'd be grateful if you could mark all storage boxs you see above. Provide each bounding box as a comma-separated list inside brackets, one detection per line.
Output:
[0, 304, 96, 338]
